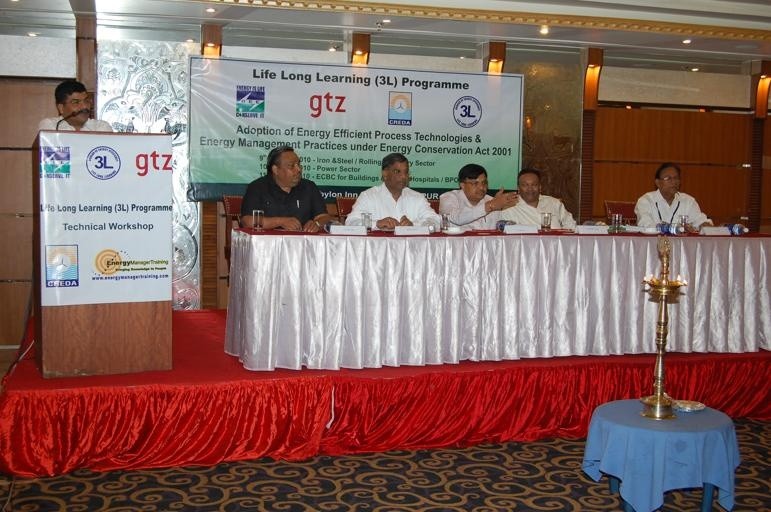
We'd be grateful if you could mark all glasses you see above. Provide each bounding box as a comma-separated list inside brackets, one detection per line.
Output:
[464, 180, 488, 186]
[66, 98, 93, 104]
[660, 176, 680, 181]
[391, 169, 409, 174]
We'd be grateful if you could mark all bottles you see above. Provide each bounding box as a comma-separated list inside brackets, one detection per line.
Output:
[583, 220, 607, 227]
[721, 222, 751, 235]
[658, 223, 686, 235]
[422, 222, 436, 233]
[496, 220, 516, 229]
[323, 219, 341, 233]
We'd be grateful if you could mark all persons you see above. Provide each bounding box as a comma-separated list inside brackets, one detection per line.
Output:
[38, 80, 113, 131]
[438, 165, 520, 230]
[502, 168, 576, 229]
[345, 153, 441, 231]
[241, 146, 330, 232]
[635, 163, 714, 234]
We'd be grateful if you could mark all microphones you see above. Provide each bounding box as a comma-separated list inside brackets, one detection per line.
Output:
[56, 107, 89, 131]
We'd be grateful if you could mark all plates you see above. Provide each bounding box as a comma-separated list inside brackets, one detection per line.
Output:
[670, 400, 706, 413]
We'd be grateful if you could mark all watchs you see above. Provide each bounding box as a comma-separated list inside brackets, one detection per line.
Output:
[488, 199, 494, 211]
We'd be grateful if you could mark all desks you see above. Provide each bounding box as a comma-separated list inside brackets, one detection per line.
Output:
[31, 125, 173, 377]
[583, 398, 736, 512]
[223, 225, 769, 371]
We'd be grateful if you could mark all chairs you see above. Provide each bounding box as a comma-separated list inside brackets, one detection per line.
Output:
[220, 195, 253, 292]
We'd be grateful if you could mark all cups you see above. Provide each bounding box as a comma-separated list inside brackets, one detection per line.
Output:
[541, 213, 552, 233]
[440, 214, 450, 234]
[252, 210, 264, 232]
[361, 213, 372, 234]
[679, 215, 689, 232]
[612, 214, 622, 234]
